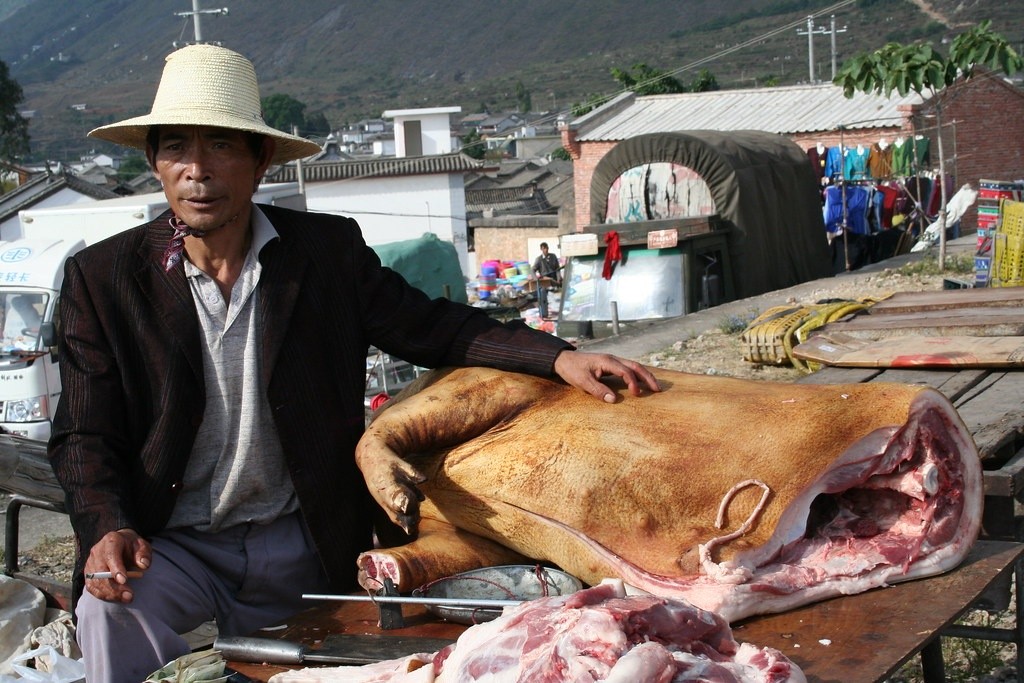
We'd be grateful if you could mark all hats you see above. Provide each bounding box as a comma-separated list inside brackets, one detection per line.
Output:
[86, 45, 322, 165]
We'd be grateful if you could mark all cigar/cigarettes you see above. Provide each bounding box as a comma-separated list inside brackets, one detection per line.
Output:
[87, 570, 143, 579]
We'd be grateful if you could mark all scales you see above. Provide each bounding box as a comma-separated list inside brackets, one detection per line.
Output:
[300, 563, 585, 626]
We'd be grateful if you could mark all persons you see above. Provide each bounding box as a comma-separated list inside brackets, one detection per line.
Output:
[44, 44, 662, 683]
[530, 242, 563, 283]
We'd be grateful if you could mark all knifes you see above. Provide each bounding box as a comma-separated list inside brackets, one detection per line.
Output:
[211, 635, 460, 666]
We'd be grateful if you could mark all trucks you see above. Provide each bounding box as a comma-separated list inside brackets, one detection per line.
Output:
[536, 130, 835, 345]
[0, 183, 303, 443]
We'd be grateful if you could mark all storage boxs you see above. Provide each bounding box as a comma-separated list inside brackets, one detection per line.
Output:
[975, 177, 1023, 287]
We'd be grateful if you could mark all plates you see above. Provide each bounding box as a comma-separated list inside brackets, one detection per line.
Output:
[414, 564, 582, 623]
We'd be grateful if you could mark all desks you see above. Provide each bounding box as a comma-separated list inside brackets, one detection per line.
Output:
[212, 538, 1024, 683]
[774, 288, 1024, 544]
